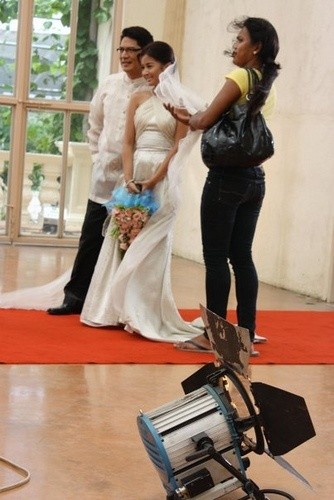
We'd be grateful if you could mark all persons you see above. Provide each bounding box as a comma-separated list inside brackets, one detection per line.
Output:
[81, 42, 191, 342]
[162, 16, 282, 355]
[45, 27, 154, 316]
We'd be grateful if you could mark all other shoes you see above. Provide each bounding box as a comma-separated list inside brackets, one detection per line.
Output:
[47, 304, 82, 314]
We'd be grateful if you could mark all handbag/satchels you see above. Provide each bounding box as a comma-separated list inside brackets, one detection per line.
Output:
[200, 66, 274, 170]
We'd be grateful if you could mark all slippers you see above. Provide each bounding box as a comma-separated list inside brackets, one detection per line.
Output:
[173, 340, 214, 353]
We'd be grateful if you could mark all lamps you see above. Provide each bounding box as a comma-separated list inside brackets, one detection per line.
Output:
[135, 305, 316, 500]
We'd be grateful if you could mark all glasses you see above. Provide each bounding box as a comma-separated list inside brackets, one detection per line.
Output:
[116, 46, 143, 54]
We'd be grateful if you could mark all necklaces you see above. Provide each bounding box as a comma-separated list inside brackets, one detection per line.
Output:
[152, 88, 154, 96]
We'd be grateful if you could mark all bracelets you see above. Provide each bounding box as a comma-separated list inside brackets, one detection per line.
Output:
[126, 179, 134, 188]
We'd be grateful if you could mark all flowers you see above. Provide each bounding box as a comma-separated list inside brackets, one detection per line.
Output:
[111, 183, 152, 253]
